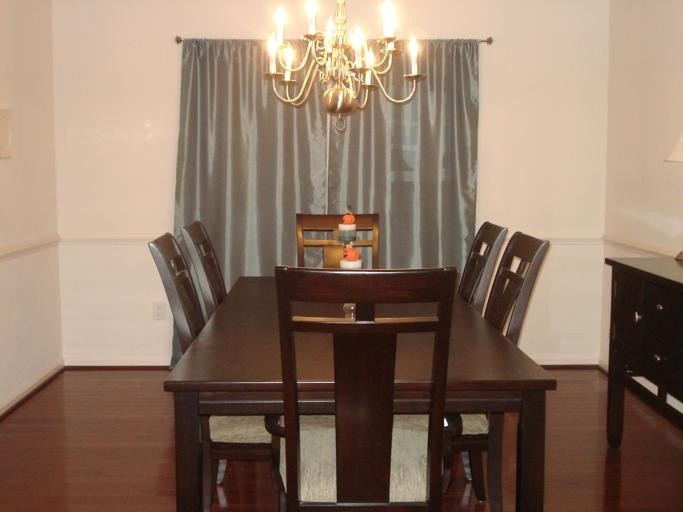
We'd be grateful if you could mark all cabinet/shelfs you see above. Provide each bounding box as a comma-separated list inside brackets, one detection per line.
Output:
[604, 256, 682, 447]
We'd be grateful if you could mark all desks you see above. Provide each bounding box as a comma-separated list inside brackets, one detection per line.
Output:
[162, 275, 556, 511]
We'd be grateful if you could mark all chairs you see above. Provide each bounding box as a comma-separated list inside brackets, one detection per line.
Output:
[179, 220, 227, 319]
[442, 231, 549, 511]
[295, 213, 378, 270]
[147, 233, 271, 512]
[443, 222, 507, 495]
[264, 265, 458, 511]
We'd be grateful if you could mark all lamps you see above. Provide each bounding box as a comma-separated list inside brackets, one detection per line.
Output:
[663, 132, 683, 261]
[264, 1, 426, 134]
